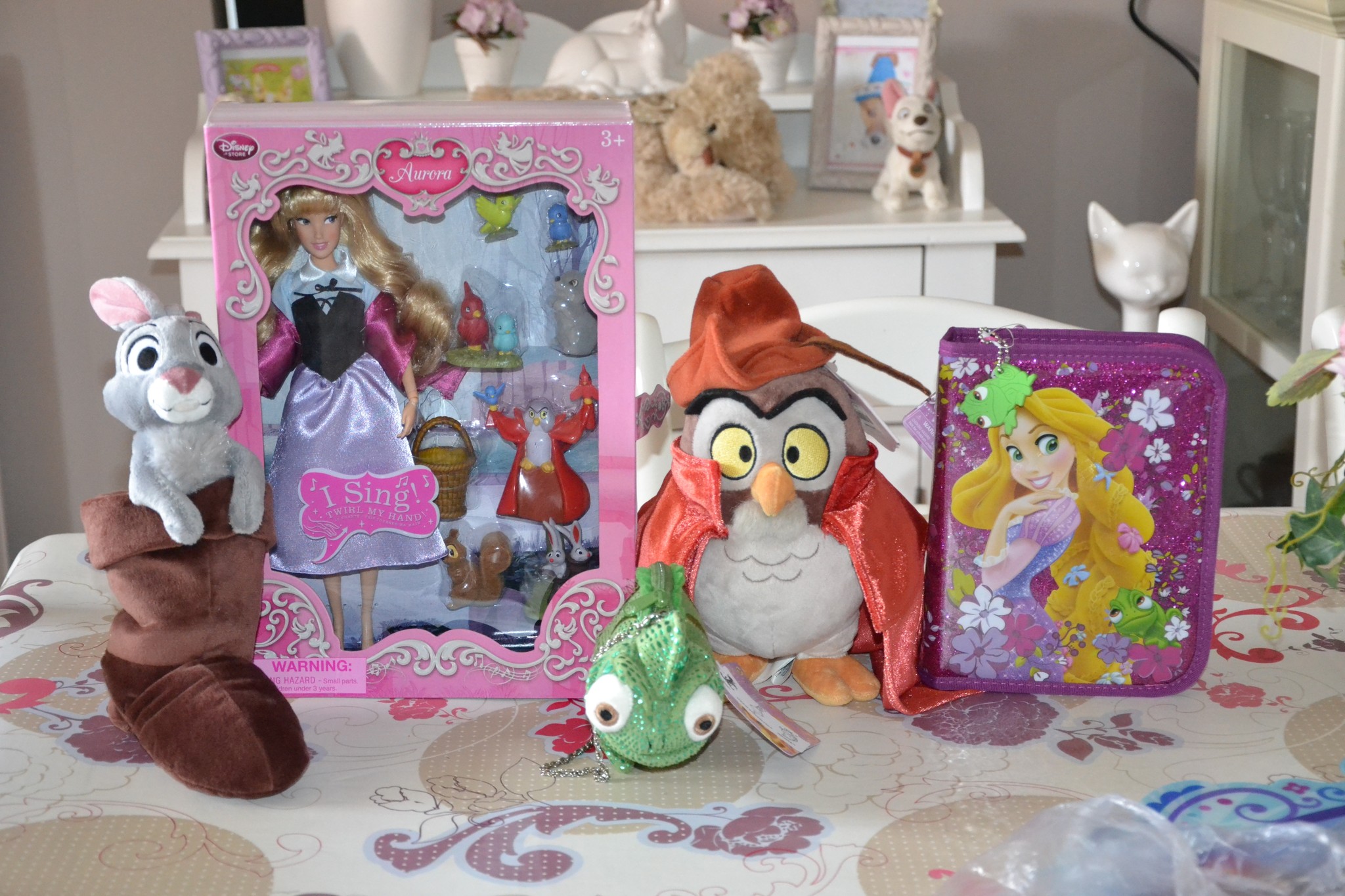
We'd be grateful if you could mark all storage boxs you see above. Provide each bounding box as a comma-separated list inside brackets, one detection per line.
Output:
[205, 96, 637, 697]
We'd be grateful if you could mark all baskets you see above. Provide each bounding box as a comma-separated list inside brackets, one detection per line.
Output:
[412, 416, 477, 519]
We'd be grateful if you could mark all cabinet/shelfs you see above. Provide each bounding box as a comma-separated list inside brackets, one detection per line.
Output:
[1184, 0, 1345, 508]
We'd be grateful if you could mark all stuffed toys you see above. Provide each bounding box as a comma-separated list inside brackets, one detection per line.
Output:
[872, 78, 947, 213]
[584, 563, 724, 773]
[629, 54, 796, 224]
[637, 266, 987, 716]
[89, 275, 265, 546]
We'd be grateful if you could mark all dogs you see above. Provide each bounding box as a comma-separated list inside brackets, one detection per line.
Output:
[869, 78, 949, 213]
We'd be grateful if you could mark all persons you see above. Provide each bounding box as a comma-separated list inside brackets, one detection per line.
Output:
[250, 188, 455, 651]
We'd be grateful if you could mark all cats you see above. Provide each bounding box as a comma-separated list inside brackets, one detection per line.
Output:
[1086, 198, 1200, 329]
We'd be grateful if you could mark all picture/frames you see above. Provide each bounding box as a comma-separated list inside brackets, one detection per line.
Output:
[808, 13, 937, 189]
[199, 26, 332, 101]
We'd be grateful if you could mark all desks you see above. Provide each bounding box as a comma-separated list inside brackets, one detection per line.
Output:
[147, 203, 1025, 342]
[0, 509, 1345, 896]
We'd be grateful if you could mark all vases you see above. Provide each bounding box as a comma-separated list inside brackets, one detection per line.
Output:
[454, 36, 522, 93]
[730, 31, 799, 88]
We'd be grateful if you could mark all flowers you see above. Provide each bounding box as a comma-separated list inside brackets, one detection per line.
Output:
[721, 0, 800, 42]
[445, 0, 527, 55]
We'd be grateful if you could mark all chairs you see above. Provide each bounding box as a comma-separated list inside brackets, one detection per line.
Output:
[637, 299, 1208, 507]
